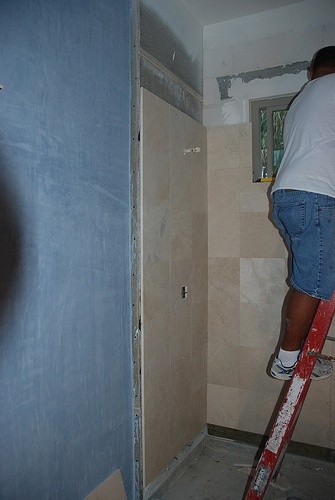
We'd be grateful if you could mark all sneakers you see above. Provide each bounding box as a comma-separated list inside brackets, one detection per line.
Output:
[269, 354, 333, 381]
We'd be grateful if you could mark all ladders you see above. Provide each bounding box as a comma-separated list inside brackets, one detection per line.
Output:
[241, 289, 335, 500]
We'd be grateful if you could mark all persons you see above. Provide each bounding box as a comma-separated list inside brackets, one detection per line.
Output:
[270, 46, 335, 381]
